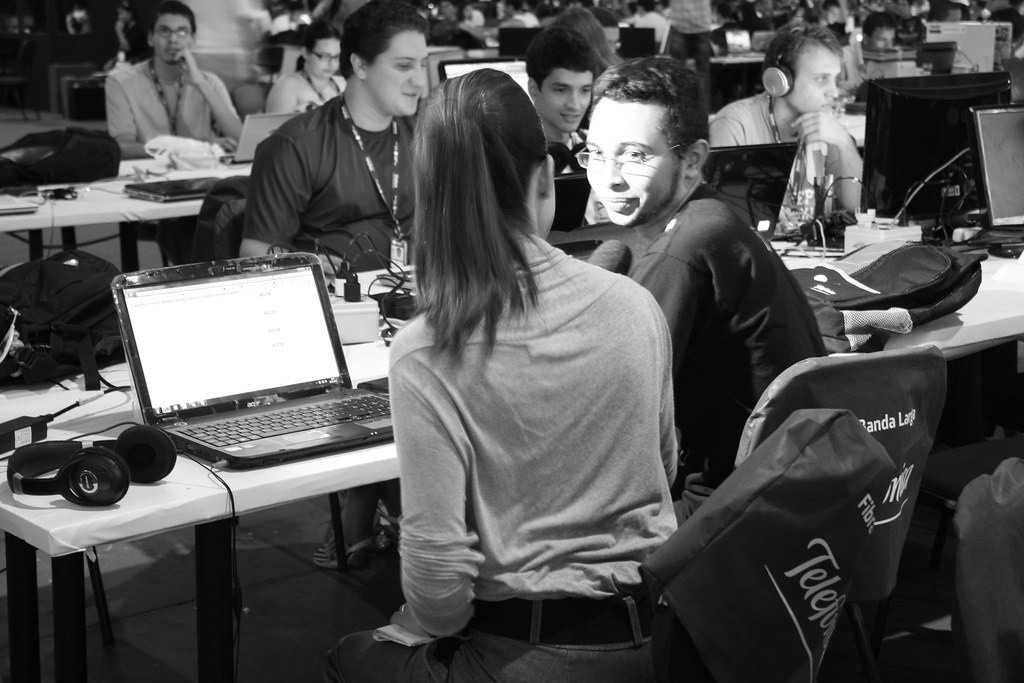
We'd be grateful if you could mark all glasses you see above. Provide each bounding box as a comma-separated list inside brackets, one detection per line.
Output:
[312, 50, 341, 64]
[153, 26, 189, 38]
[574, 144, 680, 175]
[41, 187, 77, 200]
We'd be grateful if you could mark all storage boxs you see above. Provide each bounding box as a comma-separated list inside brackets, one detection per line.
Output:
[49, 62, 100, 120]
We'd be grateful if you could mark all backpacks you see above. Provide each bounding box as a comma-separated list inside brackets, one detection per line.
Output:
[788, 243, 989, 353]
[0, 127, 122, 186]
[0, 249, 127, 392]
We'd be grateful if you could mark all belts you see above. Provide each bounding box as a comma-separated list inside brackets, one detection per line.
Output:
[468, 597, 654, 645]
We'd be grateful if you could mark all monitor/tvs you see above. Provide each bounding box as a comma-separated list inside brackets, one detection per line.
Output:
[438, 58, 534, 107]
[912, 41, 958, 76]
[859, 77, 1024, 227]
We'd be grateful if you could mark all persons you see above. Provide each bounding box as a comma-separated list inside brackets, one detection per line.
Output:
[238, 0, 434, 275]
[101, 0, 154, 71]
[841, 11, 895, 92]
[665, 0, 724, 129]
[244, 0, 672, 82]
[526, 26, 597, 179]
[64, 0, 94, 36]
[103, 0, 243, 269]
[709, 0, 1024, 55]
[299, 68, 683, 683]
[712, 18, 865, 235]
[264, 21, 346, 116]
[582, 56, 828, 531]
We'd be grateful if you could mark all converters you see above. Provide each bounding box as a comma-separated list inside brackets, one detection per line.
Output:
[372, 292, 422, 321]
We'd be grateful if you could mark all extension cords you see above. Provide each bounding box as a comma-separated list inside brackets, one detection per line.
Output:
[328, 293, 380, 342]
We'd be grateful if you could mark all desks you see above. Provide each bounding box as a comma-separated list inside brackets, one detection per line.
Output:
[1, 157, 253, 277]
[0, 221, 1024, 683]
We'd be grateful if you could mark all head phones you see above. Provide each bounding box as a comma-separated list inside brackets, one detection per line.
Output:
[762, 34, 793, 98]
[5, 425, 178, 506]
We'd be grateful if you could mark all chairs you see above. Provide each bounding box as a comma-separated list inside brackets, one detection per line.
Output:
[653, 341, 1024, 683]
[191, 174, 252, 264]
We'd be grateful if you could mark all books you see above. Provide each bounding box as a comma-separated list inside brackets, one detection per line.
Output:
[862, 49, 918, 63]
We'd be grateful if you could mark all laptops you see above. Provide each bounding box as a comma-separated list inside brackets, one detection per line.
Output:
[112, 252, 396, 470]
[696, 141, 802, 243]
[124, 176, 222, 203]
[219, 111, 303, 163]
[0, 195, 39, 214]
